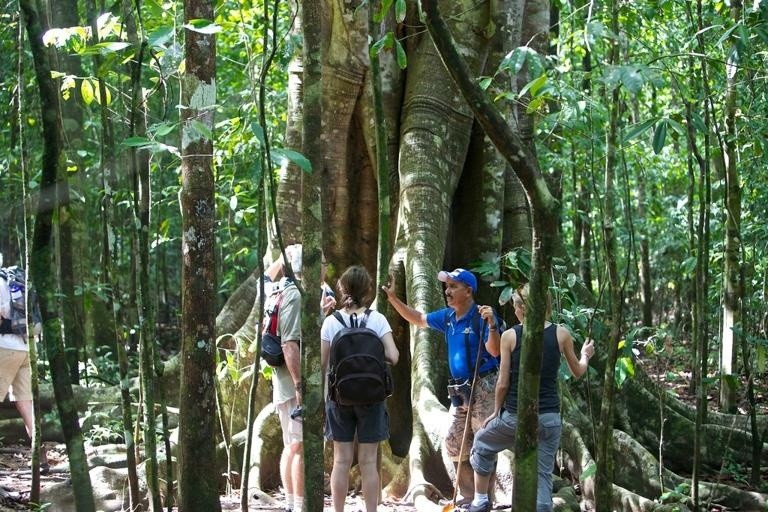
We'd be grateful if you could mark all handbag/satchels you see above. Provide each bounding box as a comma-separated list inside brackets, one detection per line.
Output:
[259, 331, 286, 368]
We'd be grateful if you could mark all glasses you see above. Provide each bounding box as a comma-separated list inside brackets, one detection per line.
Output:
[516, 284, 525, 304]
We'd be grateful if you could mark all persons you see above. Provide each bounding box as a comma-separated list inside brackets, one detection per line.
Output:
[255, 244, 335, 494]
[315, 265, 400, 512]
[0, 252, 50, 475]
[269, 244, 337, 511]
[380, 265, 508, 512]
[451, 282, 596, 511]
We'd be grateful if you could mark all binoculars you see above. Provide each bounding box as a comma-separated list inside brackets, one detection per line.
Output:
[447, 376, 475, 407]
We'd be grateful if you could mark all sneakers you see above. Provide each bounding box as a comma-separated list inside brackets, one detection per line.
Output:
[464, 500, 491, 512]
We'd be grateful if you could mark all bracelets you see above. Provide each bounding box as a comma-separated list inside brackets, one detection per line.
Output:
[488, 323, 498, 331]
[293, 381, 302, 392]
[580, 352, 592, 361]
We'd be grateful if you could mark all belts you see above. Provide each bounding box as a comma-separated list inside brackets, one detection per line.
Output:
[469, 365, 499, 383]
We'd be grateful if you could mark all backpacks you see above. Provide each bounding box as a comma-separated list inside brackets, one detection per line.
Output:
[327, 309, 397, 408]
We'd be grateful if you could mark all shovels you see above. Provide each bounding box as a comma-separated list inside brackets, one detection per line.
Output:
[441, 316, 487, 512]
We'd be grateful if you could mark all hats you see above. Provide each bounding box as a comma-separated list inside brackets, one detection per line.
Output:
[437, 267, 478, 292]
[277, 243, 302, 274]
